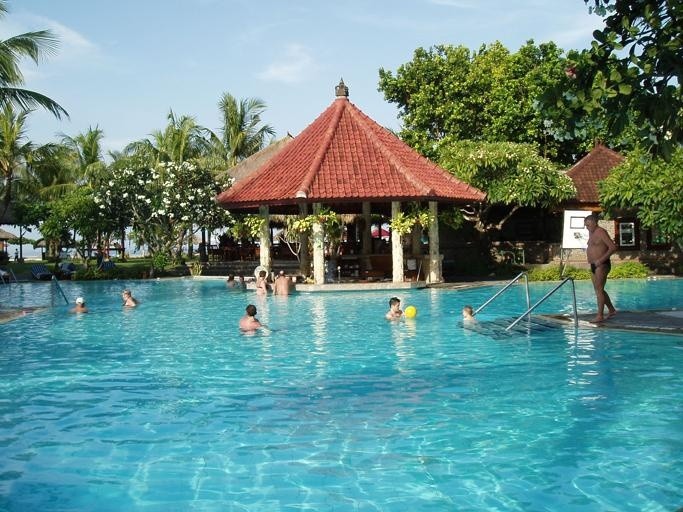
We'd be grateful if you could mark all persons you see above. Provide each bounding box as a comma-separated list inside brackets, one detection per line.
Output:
[385, 297, 404, 320]
[273, 270, 292, 295]
[122, 289, 139, 306]
[239, 304, 271, 336]
[227, 271, 269, 295]
[69, 297, 88, 313]
[462, 305, 475, 321]
[14, 249, 20, 264]
[584, 215, 618, 323]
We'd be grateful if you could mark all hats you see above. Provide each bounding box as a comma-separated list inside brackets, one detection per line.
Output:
[76, 298, 84, 304]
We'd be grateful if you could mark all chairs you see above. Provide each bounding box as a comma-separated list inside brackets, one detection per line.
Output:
[102, 262, 117, 269]
[58, 262, 77, 280]
[29, 264, 53, 280]
